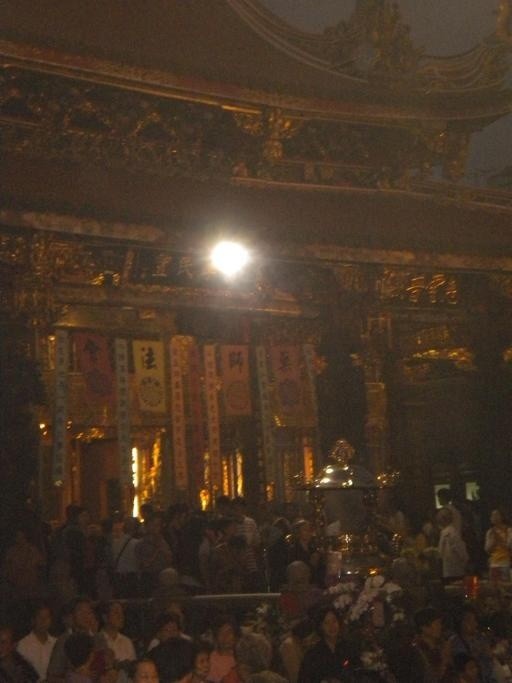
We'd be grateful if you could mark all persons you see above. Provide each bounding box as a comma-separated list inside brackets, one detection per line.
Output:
[0, 488, 512, 683]
